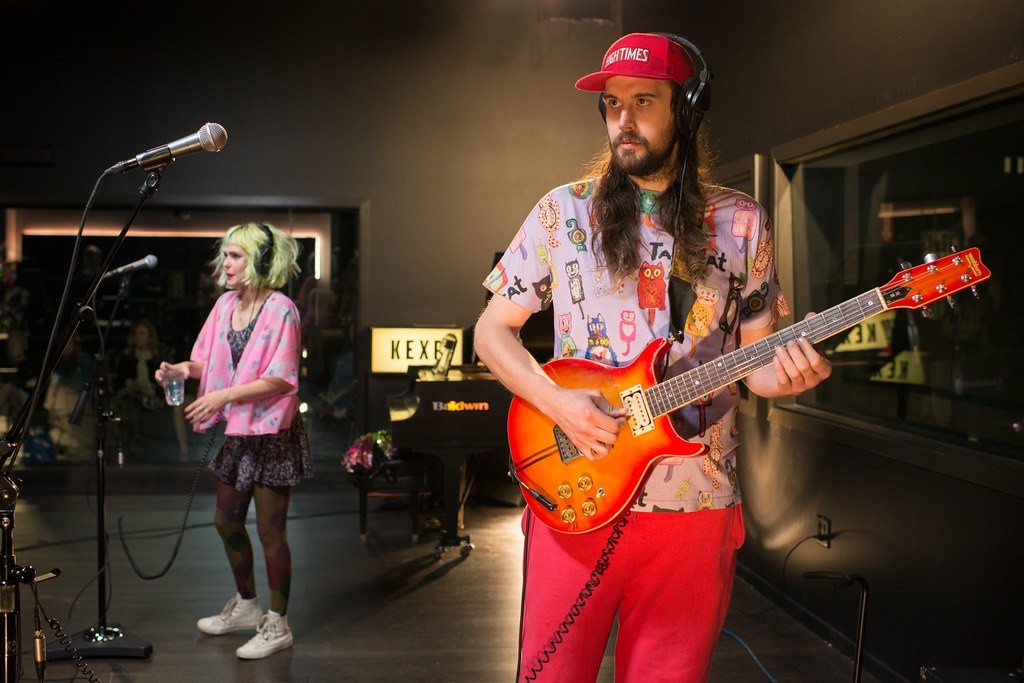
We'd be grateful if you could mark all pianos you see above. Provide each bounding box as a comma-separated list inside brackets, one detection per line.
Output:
[386, 332, 515, 563]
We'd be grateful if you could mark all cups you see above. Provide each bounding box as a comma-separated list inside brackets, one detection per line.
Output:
[162, 369, 185, 406]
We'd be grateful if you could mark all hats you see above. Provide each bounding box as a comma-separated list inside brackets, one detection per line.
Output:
[575, 33, 698, 93]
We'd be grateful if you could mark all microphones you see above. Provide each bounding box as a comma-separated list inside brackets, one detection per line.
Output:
[105, 253, 159, 278]
[104, 122, 229, 177]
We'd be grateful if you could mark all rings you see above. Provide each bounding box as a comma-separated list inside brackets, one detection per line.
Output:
[199, 405, 203, 411]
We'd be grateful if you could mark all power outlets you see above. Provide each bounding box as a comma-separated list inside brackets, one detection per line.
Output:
[815, 514, 830, 548]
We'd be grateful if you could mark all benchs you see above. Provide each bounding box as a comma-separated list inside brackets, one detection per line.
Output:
[347, 456, 438, 540]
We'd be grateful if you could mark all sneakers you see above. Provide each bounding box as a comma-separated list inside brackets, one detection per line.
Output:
[197, 592, 264, 636]
[235, 609, 293, 660]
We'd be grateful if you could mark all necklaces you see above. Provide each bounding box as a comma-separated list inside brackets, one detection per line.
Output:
[239, 317, 243, 322]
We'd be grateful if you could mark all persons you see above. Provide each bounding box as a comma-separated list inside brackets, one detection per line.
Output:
[294, 251, 336, 333]
[0, 245, 224, 454]
[154, 222, 314, 660]
[474, 33, 832, 683]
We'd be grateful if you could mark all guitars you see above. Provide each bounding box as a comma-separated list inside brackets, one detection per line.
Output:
[506, 240, 992, 535]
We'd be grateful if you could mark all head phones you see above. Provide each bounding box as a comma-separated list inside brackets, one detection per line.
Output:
[228, 221, 275, 279]
[598, 33, 715, 138]
